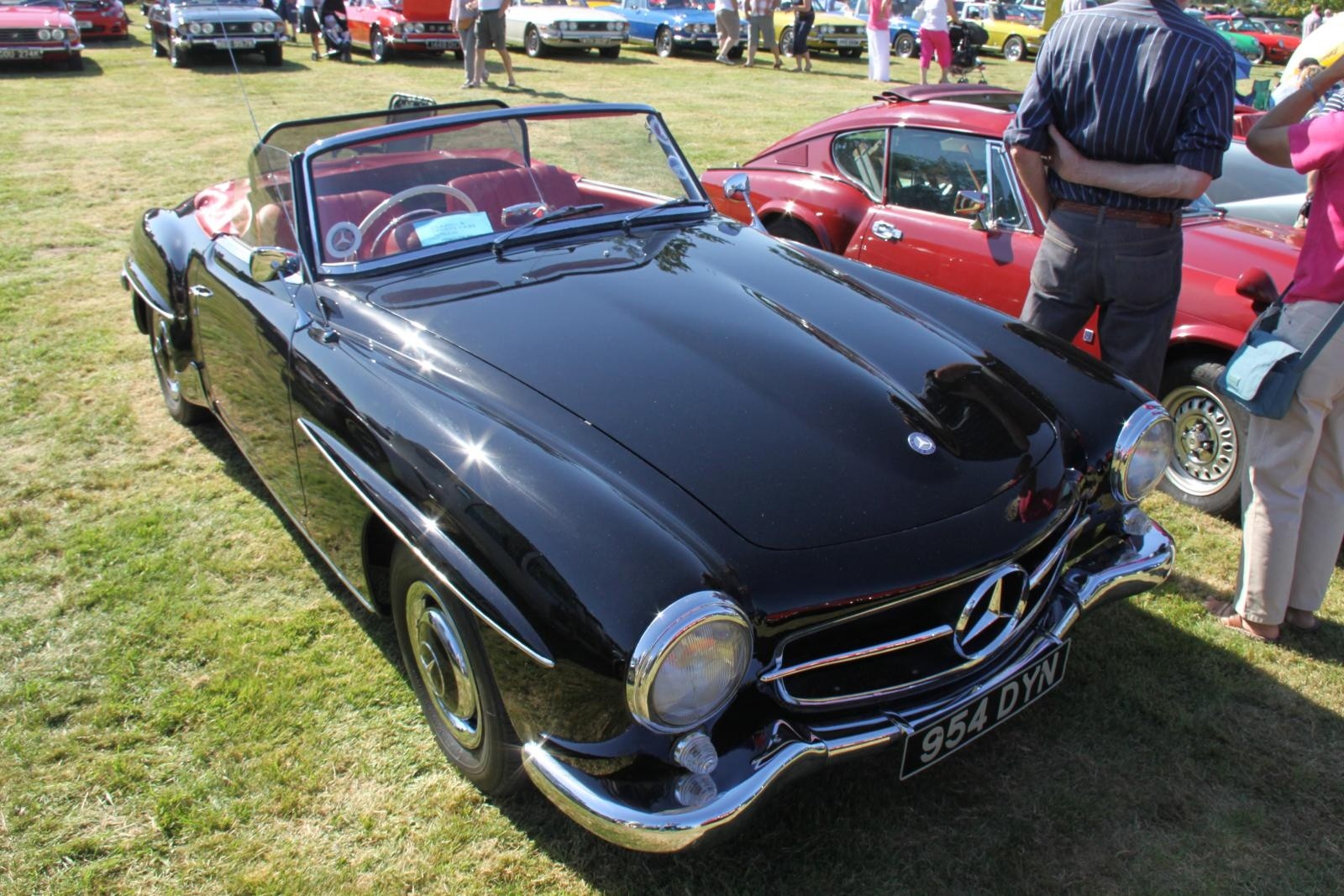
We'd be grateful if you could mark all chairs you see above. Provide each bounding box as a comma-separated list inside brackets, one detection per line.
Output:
[447, 166, 577, 246]
[268, 191, 406, 271]
[330, 94, 436, 157]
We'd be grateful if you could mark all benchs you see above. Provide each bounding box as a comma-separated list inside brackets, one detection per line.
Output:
[271, 148, 519, 207]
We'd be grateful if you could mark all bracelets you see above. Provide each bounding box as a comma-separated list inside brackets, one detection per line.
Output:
[1304, 81, 1320, 101]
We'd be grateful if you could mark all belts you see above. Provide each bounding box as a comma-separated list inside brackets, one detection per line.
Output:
[1053, 199, 1182, 225]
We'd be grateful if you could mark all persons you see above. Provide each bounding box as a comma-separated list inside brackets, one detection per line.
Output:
[1002, 0, 1236, 398]
[743, 0, 782, 69]
[790, 0, 815, 71]
[1322, 9, 1332, 22]
[920, 0, 959, 85]
[866, 0, 891, 81]
[1061, 0, 1086, 16]
[1269, 57, 1344, 119]
[1190, 3, 1257, 17]
[1202, 53, 1344, 641]
[449, 0, 489, 88]
[469, 0, 515, 87]
[263, 0, 332, 58]
[1304, 4, 1321, 38]
[714, 0, 740, 65]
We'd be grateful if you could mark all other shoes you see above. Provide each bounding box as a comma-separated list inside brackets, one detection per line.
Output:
[791, 68, 802, 71]
[461, 80, 470, 89]
[469, 80, 480, 88]
[716, 55, 734, 65]
[773, 60, 782, 70]
[741, 63, 753, 67]
[482, 73, 489, 83]
[509, 82, 515, 87]
[804, 64, 811, 72]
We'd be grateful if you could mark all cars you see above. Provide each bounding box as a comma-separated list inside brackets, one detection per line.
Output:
[504, 0, 630, 59]
[700, 82, 1308, 523]
[811, 0, 1098, 63]
[0, 0, 85, 72]
[593, 0, 749, 59]
[145, 0, 285, 67]
[65, 0, 128, 41]
[121, 97, 1177, 857]
[341, 0, 464, 64]
[737, 1, 868, 58]
[1184, 7, 1303, 65]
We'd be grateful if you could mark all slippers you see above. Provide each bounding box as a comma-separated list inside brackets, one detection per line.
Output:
[1206, 600, 1281, 643]
[1283, 616, 1320, 633]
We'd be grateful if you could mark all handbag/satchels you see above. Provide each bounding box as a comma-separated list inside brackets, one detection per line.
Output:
[1213, 329, 1304, 420]
